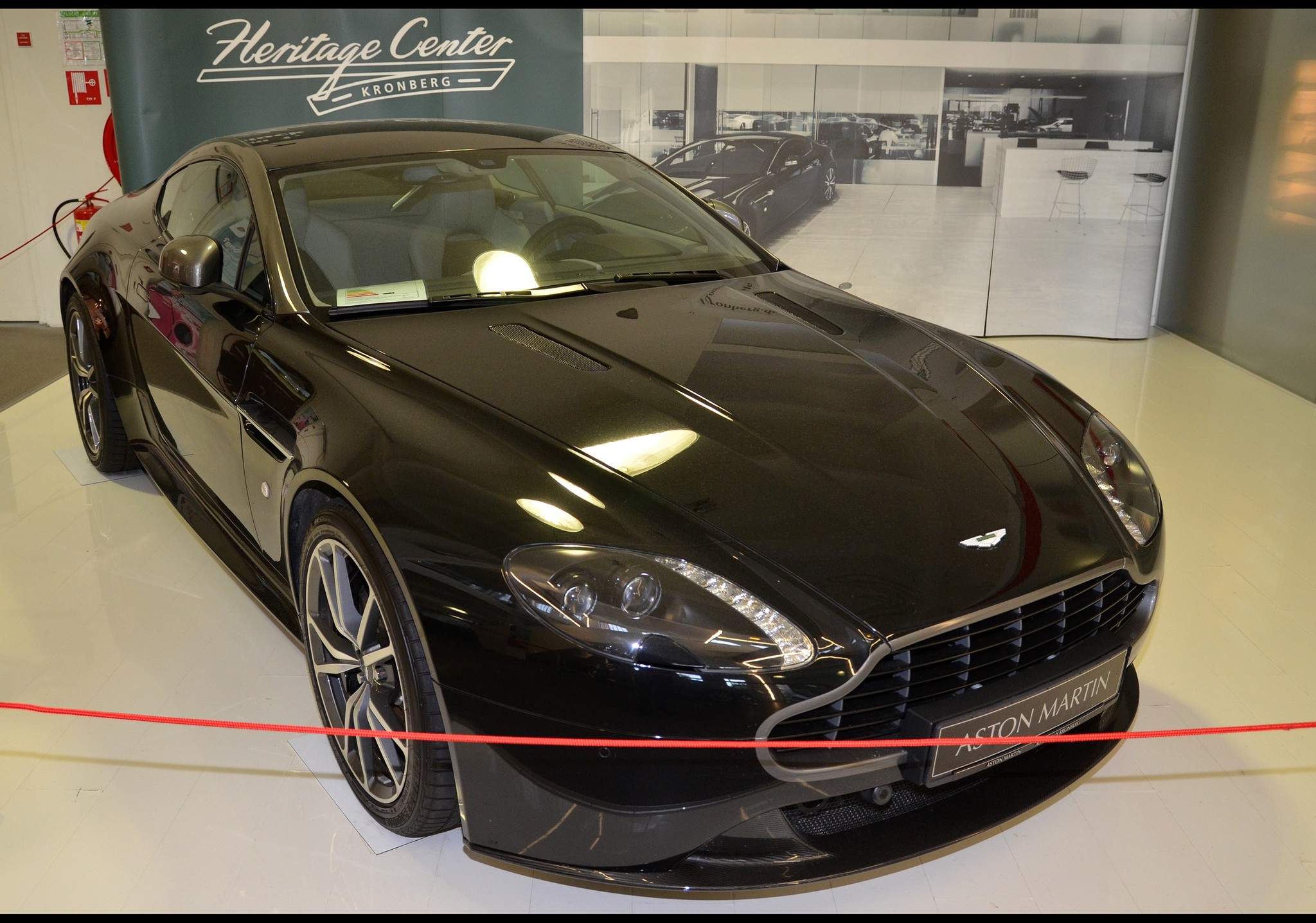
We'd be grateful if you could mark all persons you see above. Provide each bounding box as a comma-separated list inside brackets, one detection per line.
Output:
[878, 125, 900, 159]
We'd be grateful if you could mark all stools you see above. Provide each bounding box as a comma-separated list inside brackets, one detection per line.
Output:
[1117, 159, 1172, 236]
[1047, 158, 1099, 225]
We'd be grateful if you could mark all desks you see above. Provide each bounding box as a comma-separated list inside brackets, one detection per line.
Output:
[882, 146, 916, 160]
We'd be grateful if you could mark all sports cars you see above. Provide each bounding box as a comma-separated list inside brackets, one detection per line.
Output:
[54, 117, 1165, 893]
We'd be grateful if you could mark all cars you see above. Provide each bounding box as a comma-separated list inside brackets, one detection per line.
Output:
[650, 129, 838, 245]
[648, 107, 936, 161]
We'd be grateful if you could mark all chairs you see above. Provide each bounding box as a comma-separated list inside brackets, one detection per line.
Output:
[1085, 141, 1109, 149]
[408, 173, 531, 285]
[210, 171, 356, 295]
[1018, 138, 1037, 148]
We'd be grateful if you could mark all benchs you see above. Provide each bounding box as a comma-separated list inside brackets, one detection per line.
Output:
[314, 204, 420, 288]
